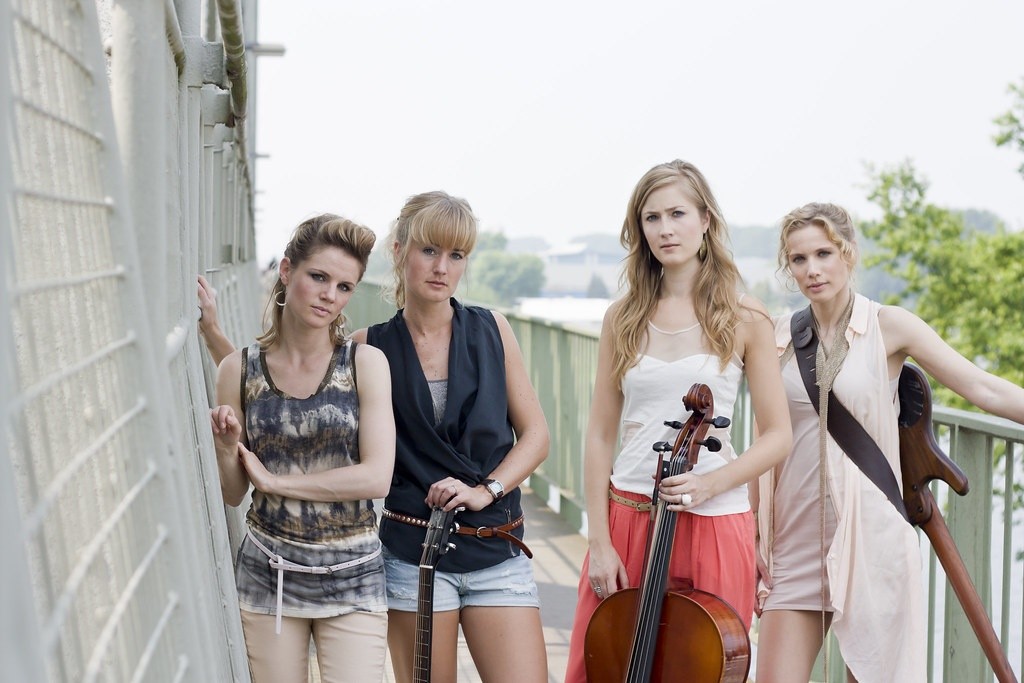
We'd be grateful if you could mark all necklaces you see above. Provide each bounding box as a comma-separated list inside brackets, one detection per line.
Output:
[649, 320, 700, 334]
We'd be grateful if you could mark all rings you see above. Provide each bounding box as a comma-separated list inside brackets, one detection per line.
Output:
[594, 587, 601, 593]
[453, 485, 458, 493]
[681, 493, 691, 505]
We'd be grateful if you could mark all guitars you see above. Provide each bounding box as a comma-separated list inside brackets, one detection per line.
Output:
[897, 361, 1024, 683]
[410, 491, 465, 683]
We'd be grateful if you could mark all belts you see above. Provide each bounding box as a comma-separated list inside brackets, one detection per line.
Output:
[381, 508, 535, 559]
[609, 489, 653, 512]
[244, 525, 381, 634]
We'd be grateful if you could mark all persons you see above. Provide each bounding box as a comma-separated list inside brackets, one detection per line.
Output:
[209, 213, 396, 683]
[197, 191, 548, 683]
[566, 159, 793, 683]
[749, 201, 1024, 683]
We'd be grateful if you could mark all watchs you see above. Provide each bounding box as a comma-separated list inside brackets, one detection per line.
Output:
[479, 479, 503, 504]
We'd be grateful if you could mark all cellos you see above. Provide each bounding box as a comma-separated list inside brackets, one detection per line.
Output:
[581, 381, 753, 683]
